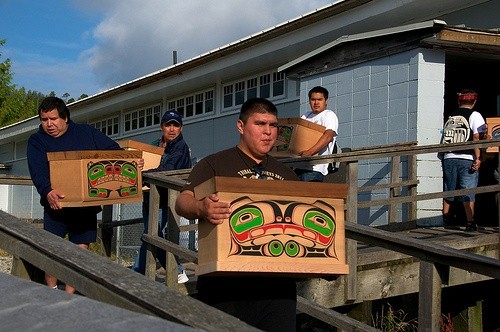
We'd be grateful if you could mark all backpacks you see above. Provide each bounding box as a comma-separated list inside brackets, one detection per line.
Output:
[306, 112, 342, 173]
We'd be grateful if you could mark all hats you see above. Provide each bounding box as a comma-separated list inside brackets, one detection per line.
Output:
[161, 110, 182, 126]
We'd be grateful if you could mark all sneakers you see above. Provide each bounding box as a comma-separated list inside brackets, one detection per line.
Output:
[178, 270, 189, 284]
[466, 222, 479, 232]
[443, 217, 451, 226]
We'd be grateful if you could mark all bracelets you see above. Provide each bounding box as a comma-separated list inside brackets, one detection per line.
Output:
[475, 157, 480, 161]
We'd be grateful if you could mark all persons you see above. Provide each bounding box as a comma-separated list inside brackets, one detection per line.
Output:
[175, 97, 339, 332]
[131, 110, 193, 284]
[27, 96, 144, 293]
[293, 86, 339, 181]
[441, 88, 486, 231]
